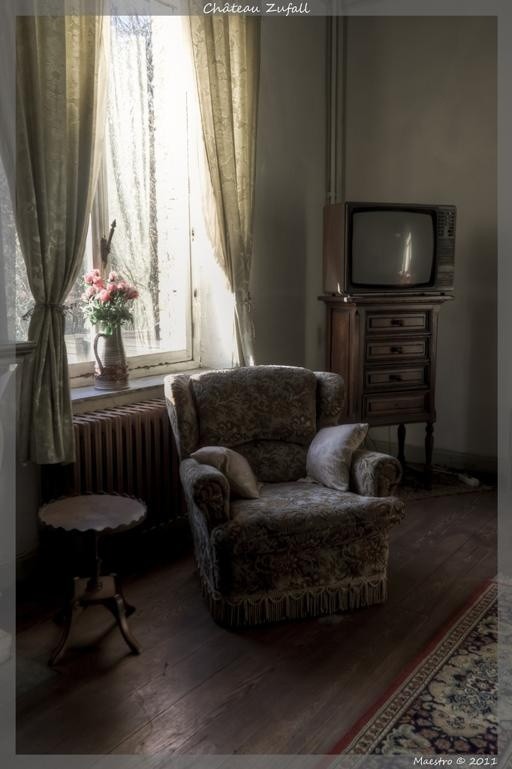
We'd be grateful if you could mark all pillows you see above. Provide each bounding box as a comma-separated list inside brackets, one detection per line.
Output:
[188, 439, 265, 499]
[301, 415, 369, 492]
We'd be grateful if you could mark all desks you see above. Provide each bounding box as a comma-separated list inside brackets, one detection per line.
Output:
[31, 490, 152, 672]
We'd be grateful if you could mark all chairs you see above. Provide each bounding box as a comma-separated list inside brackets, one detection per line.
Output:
[163, 362, 404, 632]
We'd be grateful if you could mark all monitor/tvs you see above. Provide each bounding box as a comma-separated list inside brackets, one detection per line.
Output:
[324, 202, 457, 298]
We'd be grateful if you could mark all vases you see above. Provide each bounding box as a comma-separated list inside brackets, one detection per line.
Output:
[86, 320, 133, 394]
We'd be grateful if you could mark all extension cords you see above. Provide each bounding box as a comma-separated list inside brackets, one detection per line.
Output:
[459, 471, 479, 487]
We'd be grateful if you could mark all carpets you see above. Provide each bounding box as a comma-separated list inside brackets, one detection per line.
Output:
[316, 564, 508, 768]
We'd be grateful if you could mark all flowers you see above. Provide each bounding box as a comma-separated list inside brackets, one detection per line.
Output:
[79, 262, 142, 338]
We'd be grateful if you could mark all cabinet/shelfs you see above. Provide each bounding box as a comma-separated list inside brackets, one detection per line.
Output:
[321, 296, 447, 477]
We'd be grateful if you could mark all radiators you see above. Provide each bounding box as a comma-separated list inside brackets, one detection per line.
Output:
[44, 400, 188, 534]
